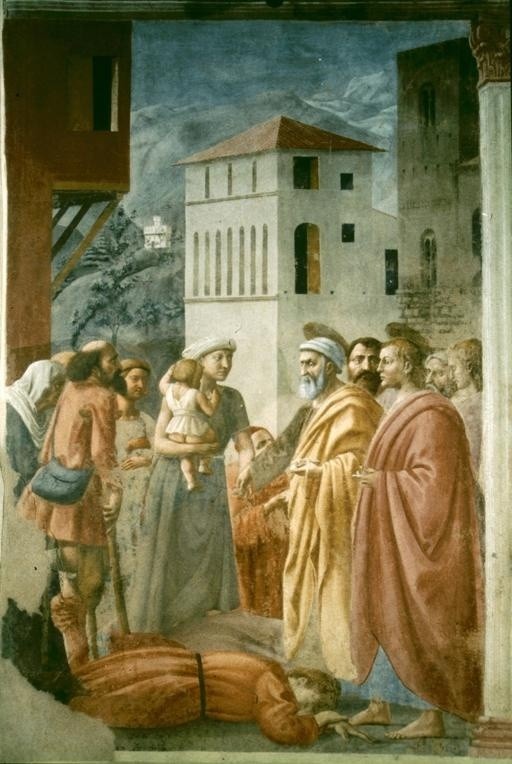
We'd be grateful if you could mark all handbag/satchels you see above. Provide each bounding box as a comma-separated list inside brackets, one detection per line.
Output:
[32, 455, 94, 505]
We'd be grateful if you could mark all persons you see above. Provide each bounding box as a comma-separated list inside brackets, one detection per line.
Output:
[342, 336, 399, 413]
[420, 348, 454, 397]
[157, 357, 220, 489]
[236, 321, 387, 691]
[45, 570, 372, 748]
[348, 322, 486, 741]
[444, 336, 483, 542]
[225, 427, 294, 620]
[5, 336, 157, 688]
[128, 334, 255, 631]
